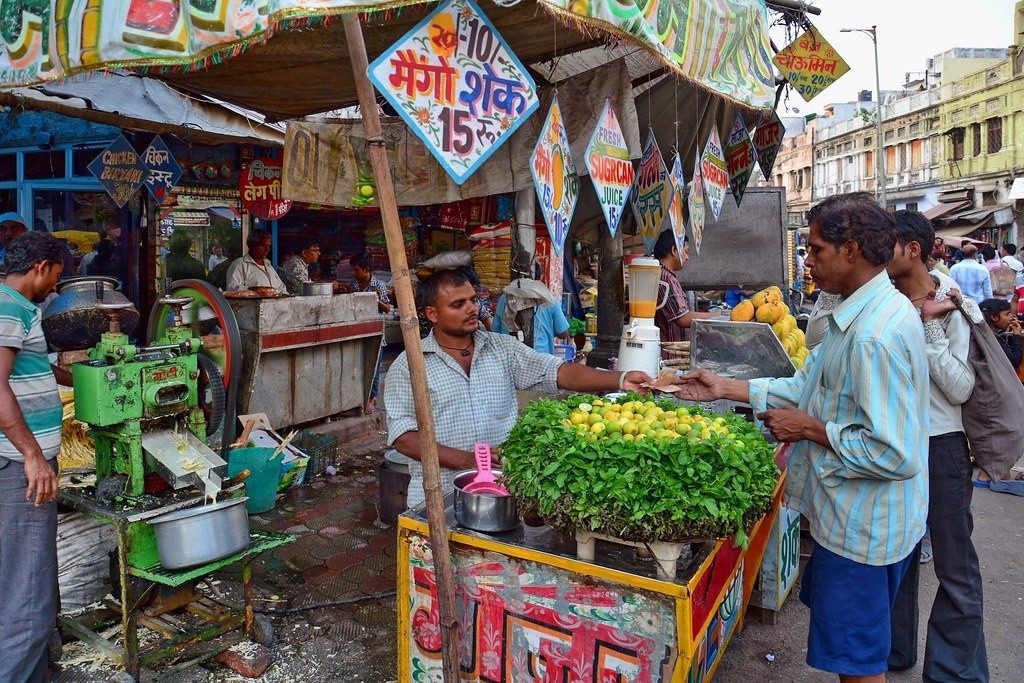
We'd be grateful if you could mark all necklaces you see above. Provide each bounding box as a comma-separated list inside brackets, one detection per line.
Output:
[438, 340, 475, 357]
[911, 295, 927, 306]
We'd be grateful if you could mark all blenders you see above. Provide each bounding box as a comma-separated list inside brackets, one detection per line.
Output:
[617, 257, 669, 383]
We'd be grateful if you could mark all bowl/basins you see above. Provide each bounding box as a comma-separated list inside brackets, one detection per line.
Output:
[453, 470, 520, 531]
[145, 497, 250, 568]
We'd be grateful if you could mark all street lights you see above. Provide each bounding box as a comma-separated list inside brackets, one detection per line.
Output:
[840, 25, 887, 211]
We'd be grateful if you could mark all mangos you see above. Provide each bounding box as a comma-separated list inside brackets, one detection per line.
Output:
[729, 285, 809, 371]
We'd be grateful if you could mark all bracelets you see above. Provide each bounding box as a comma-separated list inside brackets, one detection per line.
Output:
[619, 369, 631, 390]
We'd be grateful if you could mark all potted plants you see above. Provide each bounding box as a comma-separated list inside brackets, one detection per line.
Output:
[902, 90, 906, 98]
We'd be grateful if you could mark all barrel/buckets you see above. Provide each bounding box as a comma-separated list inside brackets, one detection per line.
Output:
[226, 448, 284, 513]
[302, 281, 334, 296]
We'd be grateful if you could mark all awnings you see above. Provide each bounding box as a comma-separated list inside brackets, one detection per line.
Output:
[921, 200, 972, 222]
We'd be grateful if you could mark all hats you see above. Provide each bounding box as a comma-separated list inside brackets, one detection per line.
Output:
[0, 212, 26, 228]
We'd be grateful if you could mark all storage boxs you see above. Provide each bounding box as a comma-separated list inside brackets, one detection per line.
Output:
[554, 344, 576, 363]
[455, 232, 471, 250]
[431, 229, 454, 251]
[585, 312, 597, 332]
[238, 412, 311, 490]
[579, 285, 596, 308]
[291, 428, 337, 478]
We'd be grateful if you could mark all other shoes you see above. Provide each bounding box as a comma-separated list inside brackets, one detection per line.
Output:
[366, 398, 377, 414]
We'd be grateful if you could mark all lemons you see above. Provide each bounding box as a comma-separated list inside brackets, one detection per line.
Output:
[564, 398, 744, 449]
[360, 185, 374, 197]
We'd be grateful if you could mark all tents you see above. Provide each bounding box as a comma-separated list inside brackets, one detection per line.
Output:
[49, 227, 108, 253]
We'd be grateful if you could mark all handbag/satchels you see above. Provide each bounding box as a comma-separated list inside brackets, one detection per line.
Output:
[951, 293, 1024, 483]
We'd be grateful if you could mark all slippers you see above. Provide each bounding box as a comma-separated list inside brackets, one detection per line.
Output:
[920, 543, 933, 563]
[972, 479, 993, 488]
[990, 476, 1024, 497]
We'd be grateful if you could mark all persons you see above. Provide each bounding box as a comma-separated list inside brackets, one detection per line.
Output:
[885, 210, 992, 683]
[208, 246, 230, 274]
[281, 236, 342, 296]
[417, 266, 495, 334]
[668, 192, 930, 683]
[380, 266, 656, 512]
[0, 211, 61, 683]
[489, 260, 571, 358]
[159, 229, 208, 281]
[625, 228, 720, 361]
[338, 249, 392, 414]
[925, 235, 1024, 498]
[36, 216, 125, 283]
[225, 229, 290, 298]
[794, 238, 815, 296]
